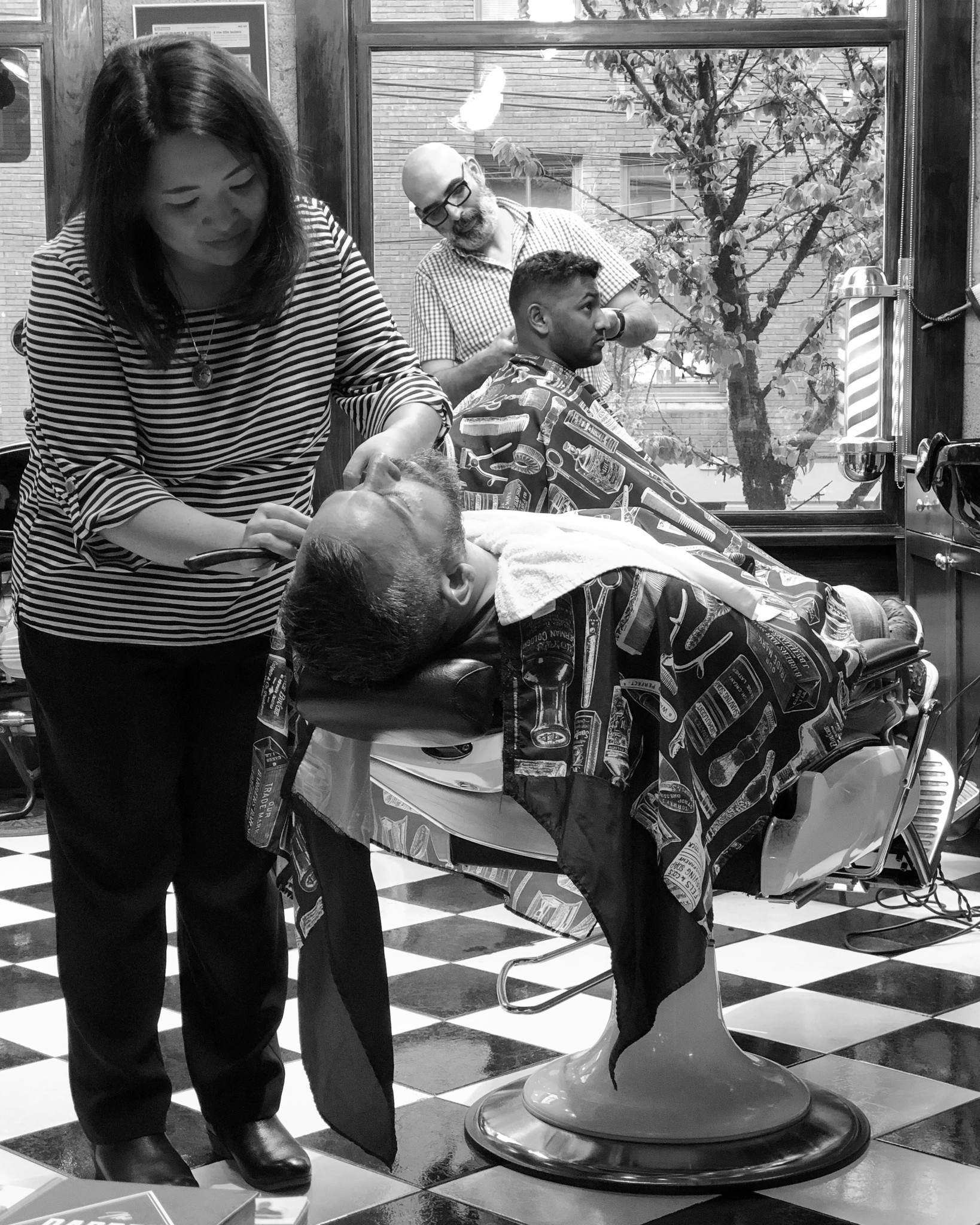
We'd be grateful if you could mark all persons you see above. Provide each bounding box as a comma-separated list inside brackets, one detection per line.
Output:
[278, 143, 940, 899]
[12, 32, 455, 1195]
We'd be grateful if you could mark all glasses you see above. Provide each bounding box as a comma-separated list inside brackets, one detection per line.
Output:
[415, 161, 472, 227]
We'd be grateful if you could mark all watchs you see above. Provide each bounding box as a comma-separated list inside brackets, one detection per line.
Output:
[603, 307, 627, 341]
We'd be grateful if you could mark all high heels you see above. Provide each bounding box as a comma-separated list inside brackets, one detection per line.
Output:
[87, 1128, 206, 1188]
[203, 1113, 312, 1190]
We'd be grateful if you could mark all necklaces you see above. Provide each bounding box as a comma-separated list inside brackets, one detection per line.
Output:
[161, 251, 227, 389]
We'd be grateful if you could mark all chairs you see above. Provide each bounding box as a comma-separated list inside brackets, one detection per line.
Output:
[251, 595, 960, 1195]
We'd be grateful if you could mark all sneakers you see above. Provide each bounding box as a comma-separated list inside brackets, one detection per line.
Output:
[886, 656, 941, 710]
[880, 596, 924, 655]
